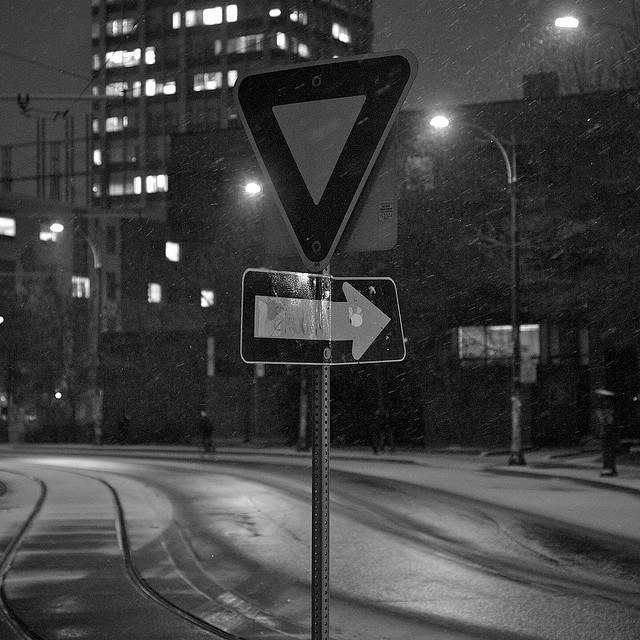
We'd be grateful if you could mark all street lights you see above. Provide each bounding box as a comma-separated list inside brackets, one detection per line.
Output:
[239, 175, 309, 456]
[550, 10, 614, 33]
[430, 116, 524, 464]
[37, 216, 109, 442]
